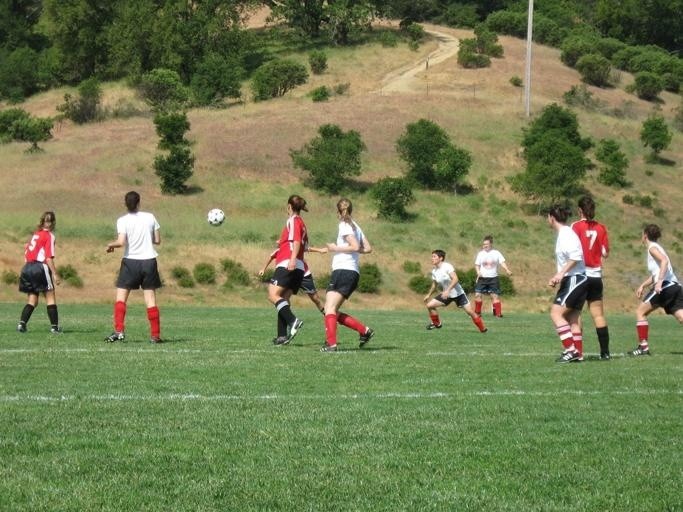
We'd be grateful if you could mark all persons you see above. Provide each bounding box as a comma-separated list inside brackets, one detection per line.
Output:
[571, 196, 612, 360]
[17, 211, 64, 334]
[627, 223, 683, 357]
[264, 195, 309, 346]
[104, 191, 162, 344]
[547, 204, 589, 363]
[473, 235, 513, 318]
[318, 198, 375, 352]
[258, 232, 330, 332]
[423, 249, 489, 333]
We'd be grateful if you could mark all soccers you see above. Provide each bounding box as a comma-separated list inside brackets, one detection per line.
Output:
[208, 208, 224, 226]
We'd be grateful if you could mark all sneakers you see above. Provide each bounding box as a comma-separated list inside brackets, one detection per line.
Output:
[599, 354, 611, 360]
[426, 322, 442, 329]
[51, 325, 63, 334]
[104, 332, 124, 343]
[284, 318, 303, 344]
[493, 314, 502, 318]
[359, 327, 375, 348]
[16, 321, 27, 332]
[320, 341, 337, 352]
[627, 345, 649, 355]
[570, 356, 584, 362]
[480, 329, 487, 332]
[150, 336, 163, 343]
[469, 314, 481, 319]
[555, 349, 579, 363]
[272, 336, 287, 345]
